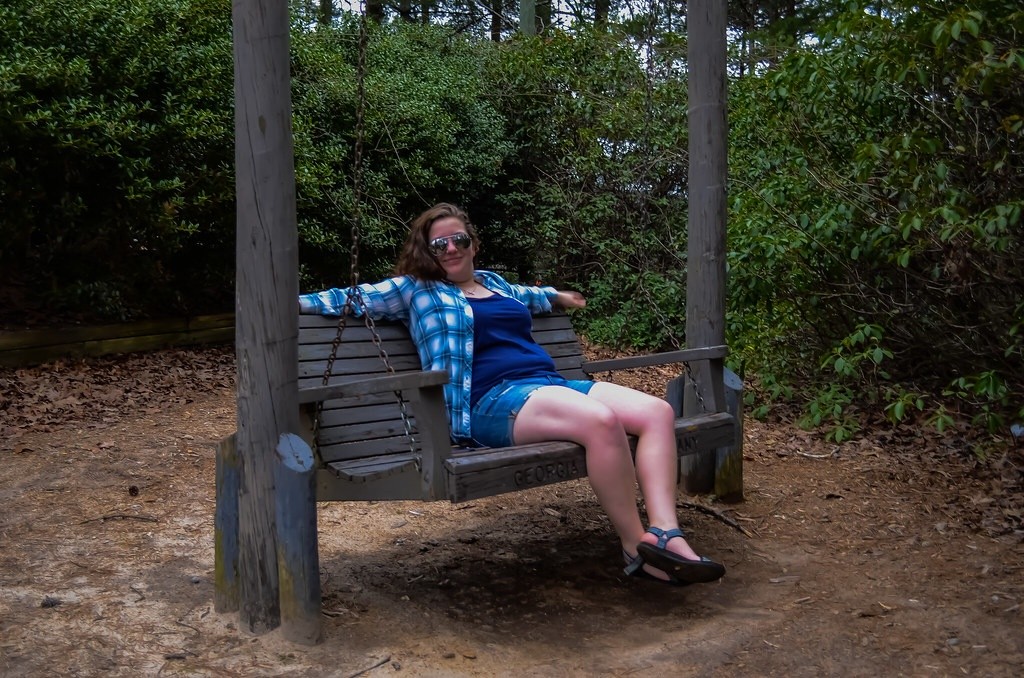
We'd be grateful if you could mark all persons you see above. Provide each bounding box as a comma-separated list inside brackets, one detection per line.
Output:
[295, 197, 728, 588]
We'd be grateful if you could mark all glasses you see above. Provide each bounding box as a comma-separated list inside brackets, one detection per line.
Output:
[429, 232, 471, 256]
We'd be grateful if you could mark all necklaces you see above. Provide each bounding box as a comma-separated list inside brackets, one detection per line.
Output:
[457, 281, 478, 295]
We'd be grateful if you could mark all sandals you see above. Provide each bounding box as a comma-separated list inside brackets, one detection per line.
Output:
[623, 526, 725, 587]
